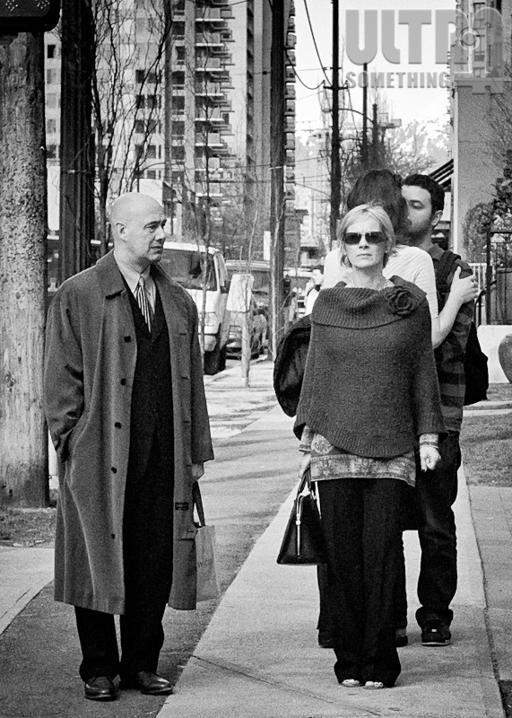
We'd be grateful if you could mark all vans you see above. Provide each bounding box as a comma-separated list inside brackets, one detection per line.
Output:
[156, 241, 236, 375]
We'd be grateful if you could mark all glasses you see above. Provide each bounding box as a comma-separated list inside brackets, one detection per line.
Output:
[343, 232, 383, 244]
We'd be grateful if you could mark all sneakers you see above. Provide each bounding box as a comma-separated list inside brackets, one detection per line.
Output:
[318, 612, 407, 688]
[420, 612, 452, 646]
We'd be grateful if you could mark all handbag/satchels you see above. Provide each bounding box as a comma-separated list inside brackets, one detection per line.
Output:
[276, 493, 321, 564]
[196, 524, 221, 604]
[435, 250, 488, 406]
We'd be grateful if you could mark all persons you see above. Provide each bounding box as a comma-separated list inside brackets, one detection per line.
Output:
[316, 168, 481, 650]
[393, 173, 478, 646]
[293, 201, 449, 693]
[304, 262, 323, 315]
[41, 191, 214, 698]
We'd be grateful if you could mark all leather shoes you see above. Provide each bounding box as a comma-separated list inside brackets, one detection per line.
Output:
[85, 675, 117, 700]
[123, 670, 174, 694]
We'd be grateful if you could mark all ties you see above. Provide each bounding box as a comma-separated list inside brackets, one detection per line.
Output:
[136, 277, 154, 332]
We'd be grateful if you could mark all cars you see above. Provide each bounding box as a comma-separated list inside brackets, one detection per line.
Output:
[221, 256, 317, 361]
